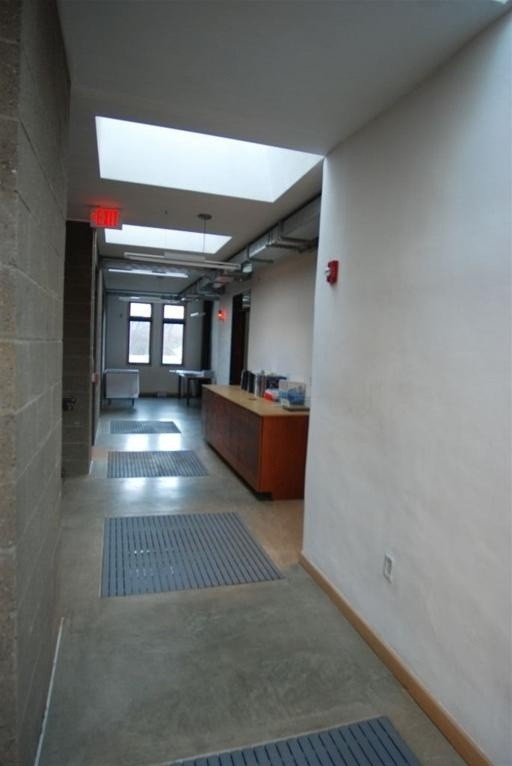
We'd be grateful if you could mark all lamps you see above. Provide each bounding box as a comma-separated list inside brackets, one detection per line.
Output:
[125, 209, 242, 273]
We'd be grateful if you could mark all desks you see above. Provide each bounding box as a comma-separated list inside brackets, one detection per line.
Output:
[171, 369, 213, 407]
[200, 383, 309, 500]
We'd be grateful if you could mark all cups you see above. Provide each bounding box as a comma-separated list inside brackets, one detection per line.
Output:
[254, 372, 266, 399]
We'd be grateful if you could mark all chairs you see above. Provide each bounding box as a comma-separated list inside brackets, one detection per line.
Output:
[106, 367, 140, 408]
